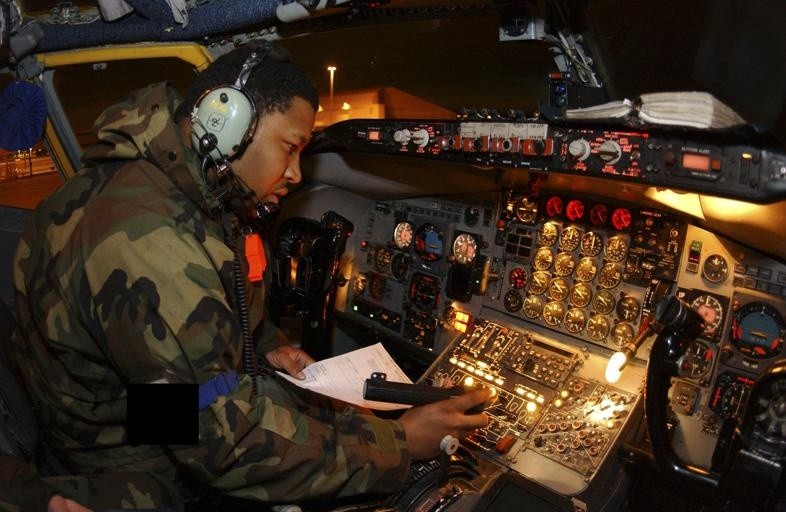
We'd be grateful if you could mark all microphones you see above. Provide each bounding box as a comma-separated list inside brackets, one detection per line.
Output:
[228, 163, 281, 224]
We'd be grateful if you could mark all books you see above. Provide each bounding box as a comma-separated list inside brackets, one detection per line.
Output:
[637, 91, 747, 131]
[0, 155, 58, 180]
[565, 98, 633, 120]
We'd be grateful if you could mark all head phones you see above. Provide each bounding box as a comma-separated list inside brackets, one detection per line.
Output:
[184, 41, 270, 162]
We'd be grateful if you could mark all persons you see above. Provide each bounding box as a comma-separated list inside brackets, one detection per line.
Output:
[13, 39, 493, 505]
[0, 451, 184, 512]
